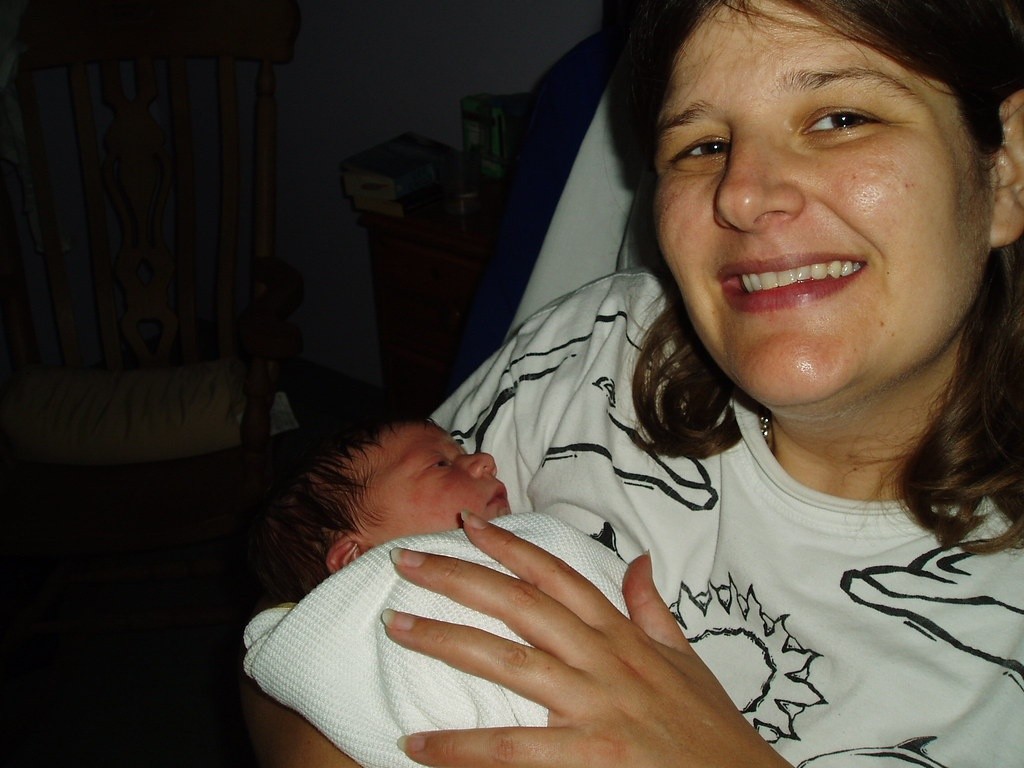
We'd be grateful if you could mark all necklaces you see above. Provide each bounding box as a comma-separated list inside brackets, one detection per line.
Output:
[760, 415, 769, 444]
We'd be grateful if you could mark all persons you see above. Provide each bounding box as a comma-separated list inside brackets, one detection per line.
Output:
[243, 413, 631, 768]
[240, 0, 1024, 768]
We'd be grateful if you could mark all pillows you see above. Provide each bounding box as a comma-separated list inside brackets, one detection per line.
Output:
[1, 359, 301, 466]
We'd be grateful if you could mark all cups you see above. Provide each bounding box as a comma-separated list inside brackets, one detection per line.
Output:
[441, 145, 481, 215]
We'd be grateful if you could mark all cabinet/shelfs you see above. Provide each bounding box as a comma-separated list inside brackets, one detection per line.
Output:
[353, 188, 508, 419]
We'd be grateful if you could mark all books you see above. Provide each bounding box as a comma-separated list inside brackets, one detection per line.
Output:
[337, 129, 480, 218]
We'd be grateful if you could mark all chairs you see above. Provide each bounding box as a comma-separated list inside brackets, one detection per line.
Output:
[446, 30, 688, 396]
[0, 0, 304, 637]
